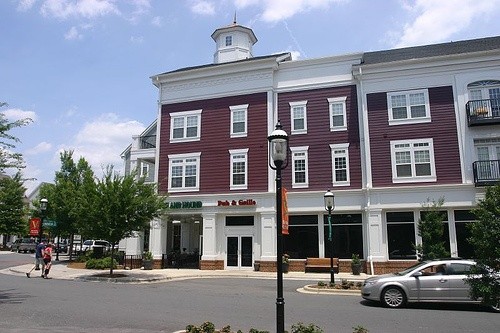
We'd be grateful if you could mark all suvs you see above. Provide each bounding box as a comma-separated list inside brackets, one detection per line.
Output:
[55, 242, 68, 253]
[82, 239, 113, 253]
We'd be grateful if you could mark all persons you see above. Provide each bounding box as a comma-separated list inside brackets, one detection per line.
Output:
[43, 243, 52, 279]
[26, 238, 47, 278]
[434, 266, 444, 275]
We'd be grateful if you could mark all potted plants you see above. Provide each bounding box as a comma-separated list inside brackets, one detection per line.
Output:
[141, 251, 154, 270]
[282, 253, 290, 273]
[350, 253, 362, 275]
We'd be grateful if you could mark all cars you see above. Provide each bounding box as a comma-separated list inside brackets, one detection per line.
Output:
[360, 257, 500, 314]
[10, 237, 36, 253]
[40, 242, 55, 248]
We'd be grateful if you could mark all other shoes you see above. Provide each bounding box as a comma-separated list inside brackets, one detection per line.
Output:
[26, 273, 30, 278]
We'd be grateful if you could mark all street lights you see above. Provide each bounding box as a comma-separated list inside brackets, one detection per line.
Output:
[266, 119, 291, 333]
[36, 198, 48, 243]
[322, 187, 337, 287]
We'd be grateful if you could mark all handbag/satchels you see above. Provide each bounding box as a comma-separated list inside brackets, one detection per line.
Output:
[45, 262, 51, 274]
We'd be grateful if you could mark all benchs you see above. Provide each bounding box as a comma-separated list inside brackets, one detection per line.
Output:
[304, 258, 339, 274]
